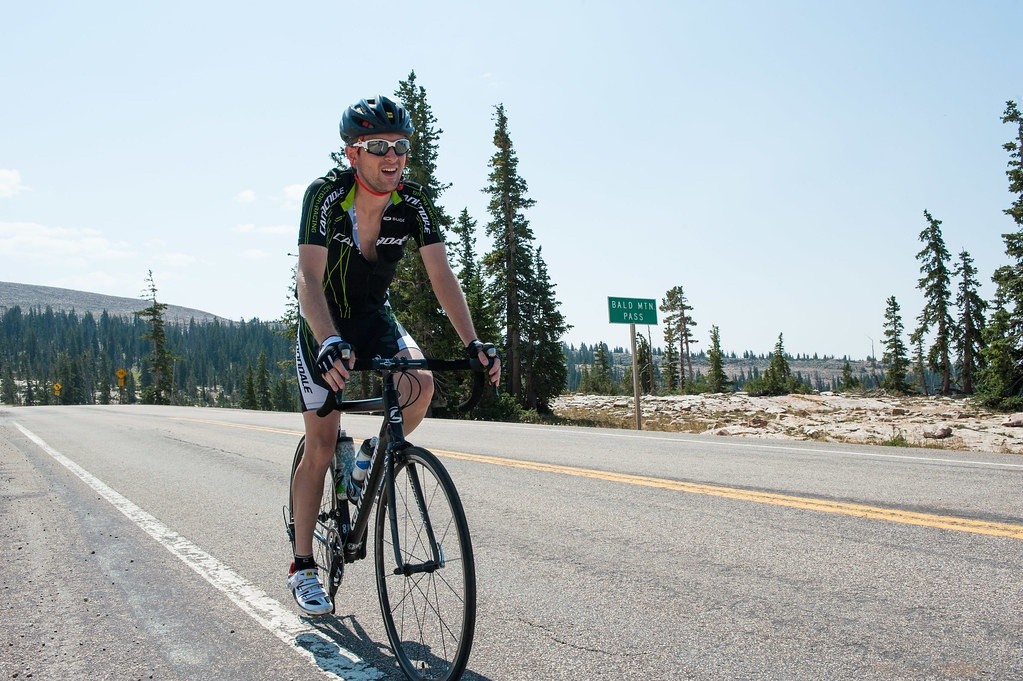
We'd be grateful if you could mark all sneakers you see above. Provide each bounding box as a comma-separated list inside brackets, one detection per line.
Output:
[286, 561, 333, 615]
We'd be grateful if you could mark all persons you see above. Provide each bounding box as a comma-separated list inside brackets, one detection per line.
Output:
[288, 94, 502, 613]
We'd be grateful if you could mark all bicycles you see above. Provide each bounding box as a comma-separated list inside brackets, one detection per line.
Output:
[282, 335, 499, 674]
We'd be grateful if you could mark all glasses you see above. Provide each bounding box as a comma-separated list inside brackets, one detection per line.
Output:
[351, 138, 411, 156]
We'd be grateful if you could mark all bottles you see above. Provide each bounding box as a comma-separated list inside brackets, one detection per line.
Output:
[347, 436, 379, 503]
[334, 430, 355, 500]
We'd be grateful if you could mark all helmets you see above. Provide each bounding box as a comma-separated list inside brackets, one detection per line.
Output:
[338, 95, 415, 142]
